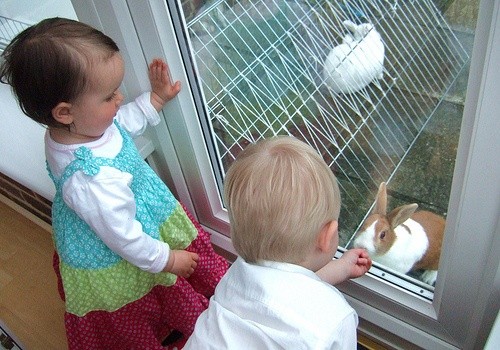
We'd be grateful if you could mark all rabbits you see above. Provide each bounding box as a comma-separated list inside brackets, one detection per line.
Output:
[322, 19, 385, 96]
[353, 182, 446, 288]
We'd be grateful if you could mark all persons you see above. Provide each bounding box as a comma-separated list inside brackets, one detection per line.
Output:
[179, 134, 372, 350]
[0, 16, 231, 350]
